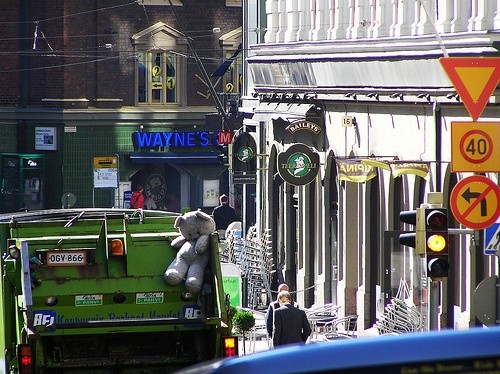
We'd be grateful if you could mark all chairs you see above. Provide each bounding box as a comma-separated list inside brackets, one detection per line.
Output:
[373, 298, 422, 335]
[236, 304, 359, 354]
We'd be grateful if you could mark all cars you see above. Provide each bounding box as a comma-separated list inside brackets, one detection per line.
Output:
[168, 327, 500, 374]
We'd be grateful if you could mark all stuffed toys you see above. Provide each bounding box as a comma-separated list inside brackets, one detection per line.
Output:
[163, 210, 216, 291]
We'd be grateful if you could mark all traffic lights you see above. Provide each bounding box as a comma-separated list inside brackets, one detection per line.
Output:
[398, 208, 424, 255]
[424, 208, 450, 278]
[216, 143, 228, 164]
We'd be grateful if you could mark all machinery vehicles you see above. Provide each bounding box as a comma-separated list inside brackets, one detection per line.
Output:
[0, 202, 238, 374]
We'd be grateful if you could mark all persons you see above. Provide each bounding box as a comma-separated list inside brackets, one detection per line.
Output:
[213, 194, 236, 238]
[168, 194, 179, 210]
[130, 186, 144, 209]
[266, 285, 300, 339]
[145, 195, 157, 209]
[274, 290, 312, 347]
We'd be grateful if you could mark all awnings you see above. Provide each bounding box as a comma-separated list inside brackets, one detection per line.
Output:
[129, 152, 217, 164]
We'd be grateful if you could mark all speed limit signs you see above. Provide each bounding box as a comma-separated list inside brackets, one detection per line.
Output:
[459, 129, 494, 164]
